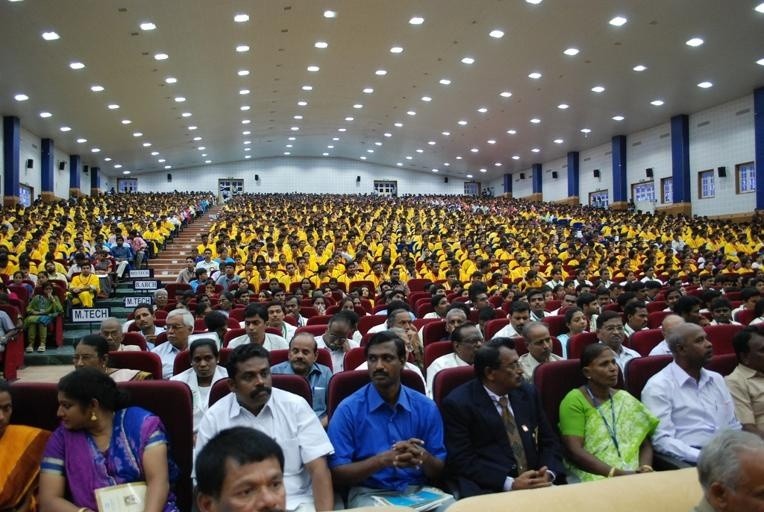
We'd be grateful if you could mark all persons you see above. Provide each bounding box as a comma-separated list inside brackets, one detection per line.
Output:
[558, 341, 660, 480]
[688, 428, 762, 511]
[36, 365, 179, 512]
[720, 328, 764, 440]
[191, 423, 289, 510]
[194, 343, 340, 511]
[2, 189, 764, 435]
[442, 336, 578, 495]
[1, 379, 55, 511]
[324, 329, 456, 511]
[638, 320, 742, 469]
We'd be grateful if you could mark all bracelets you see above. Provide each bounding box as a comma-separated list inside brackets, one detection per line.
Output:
[639, 464, 653, 472]
[606, 465, 615, 477]
[75, 506, 88, 512]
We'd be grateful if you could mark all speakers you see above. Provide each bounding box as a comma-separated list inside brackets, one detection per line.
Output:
[718, 167, 726, 177]
[594, 170, 599, 177]
[356, 176, 361, 181]
[60, 160, 66, 170]
[167, 173, 172, 180]
[26, 158, 36, 169]
[646, 168, 653, 177]
[444, 177, 449, 182]
[552, 171, 558, 178]
[83, 164, 89, 173]
[254, 175, 258, 180]
[519, 173, 525, 180]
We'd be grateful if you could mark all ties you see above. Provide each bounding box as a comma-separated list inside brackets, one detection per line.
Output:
[500, 397, 527, 473]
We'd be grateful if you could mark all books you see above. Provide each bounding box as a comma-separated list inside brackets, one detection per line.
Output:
[372, 484, 451, 509]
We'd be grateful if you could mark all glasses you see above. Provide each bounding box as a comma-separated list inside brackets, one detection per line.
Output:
[460, 337, 485, 344]
[68, 355, 98, 361]
[163, 324, 184, 330]
[327, 330, 346, 342]
[492, 361, 520, 371]
